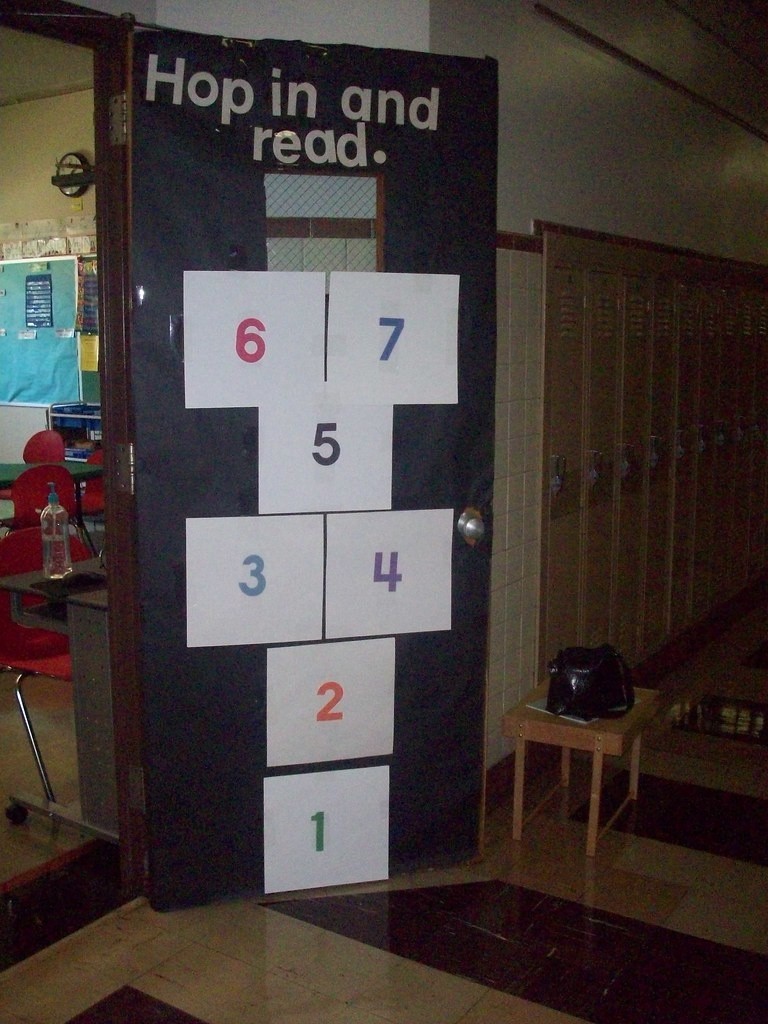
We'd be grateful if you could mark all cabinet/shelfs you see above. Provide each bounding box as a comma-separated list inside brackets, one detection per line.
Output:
[538, 230, 767, 688]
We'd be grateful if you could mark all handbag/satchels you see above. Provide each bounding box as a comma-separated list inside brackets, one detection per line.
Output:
[546, 642, 634, 721]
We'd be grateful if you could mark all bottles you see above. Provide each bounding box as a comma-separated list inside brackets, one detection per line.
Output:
[40, 482, 74, 580]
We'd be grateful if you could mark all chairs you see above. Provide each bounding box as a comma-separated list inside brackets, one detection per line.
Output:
[0, 428, 105, 805]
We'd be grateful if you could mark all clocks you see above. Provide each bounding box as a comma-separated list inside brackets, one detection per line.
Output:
[55, 152, 89, 198]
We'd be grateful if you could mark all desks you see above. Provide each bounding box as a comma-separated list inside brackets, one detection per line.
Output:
[0, 462, 106, 557]
[0, 559, 122, 845]
[0, 499, 15, 520]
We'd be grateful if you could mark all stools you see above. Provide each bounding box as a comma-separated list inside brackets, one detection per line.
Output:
[501, 677, 663, 860]
